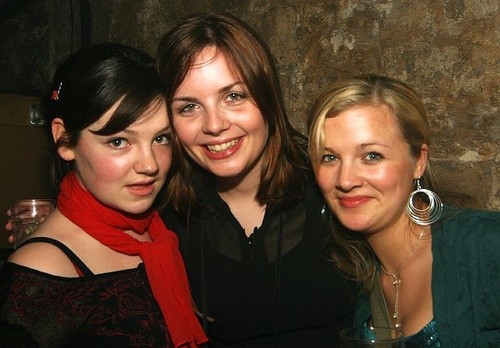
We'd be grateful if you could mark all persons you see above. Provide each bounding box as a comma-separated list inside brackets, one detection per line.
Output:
[0, 12, 500, 348]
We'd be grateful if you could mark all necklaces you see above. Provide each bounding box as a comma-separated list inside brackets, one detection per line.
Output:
[381, 226, 424, 329]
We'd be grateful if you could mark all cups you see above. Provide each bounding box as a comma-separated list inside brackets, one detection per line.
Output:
[11, 199, 53, 250]
[338, 327, 407, 348]
